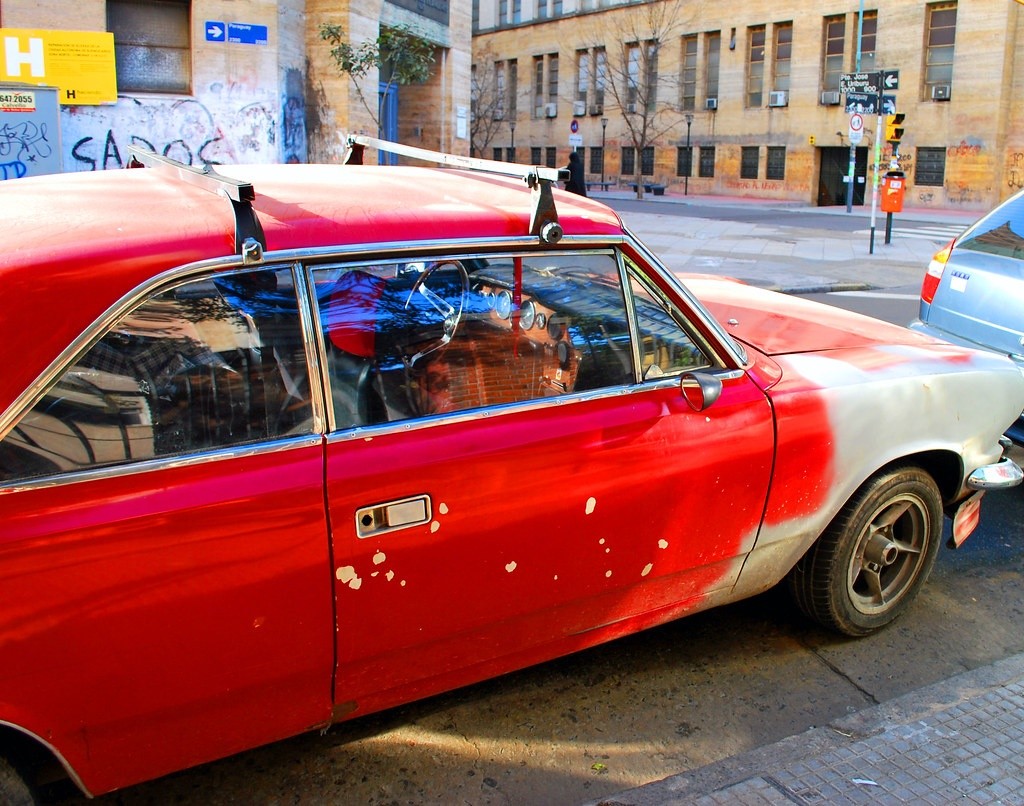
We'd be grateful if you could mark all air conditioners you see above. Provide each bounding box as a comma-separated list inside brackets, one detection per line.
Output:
[770, 91, 788, 107]
[627, 104, 637, 112]
[932, 86, 950, 98]
[821, 92, 839, 103]
[546, 103, 556, 117]
[494, 111, 503, 120]
[573, 101, 585, 116]
[706, 99, 716, 108]
[590, 105, 602, 115]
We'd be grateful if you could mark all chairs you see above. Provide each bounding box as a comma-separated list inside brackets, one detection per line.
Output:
[326, 271, 419, 431]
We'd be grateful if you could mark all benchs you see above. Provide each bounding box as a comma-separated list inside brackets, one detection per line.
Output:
[627, 183, 667, 195]
[585, 182, 616, 191]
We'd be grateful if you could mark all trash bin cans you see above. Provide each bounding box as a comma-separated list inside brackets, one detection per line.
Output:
[881, 172, 906, 212]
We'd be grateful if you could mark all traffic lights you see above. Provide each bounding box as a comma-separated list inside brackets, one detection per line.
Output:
[886, 112, 906, 143]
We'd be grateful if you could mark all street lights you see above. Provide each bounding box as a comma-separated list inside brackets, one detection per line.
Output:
[601, 118, 609, 190]
[510, 122, 516, 162]
[685, 114, 694, 195]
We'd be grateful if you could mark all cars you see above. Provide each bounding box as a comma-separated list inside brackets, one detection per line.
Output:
[907, 189, 1024, 446]
[0, 132, 1023, 805]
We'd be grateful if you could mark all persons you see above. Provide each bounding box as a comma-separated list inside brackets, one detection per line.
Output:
[562, 153, 588, 197]
